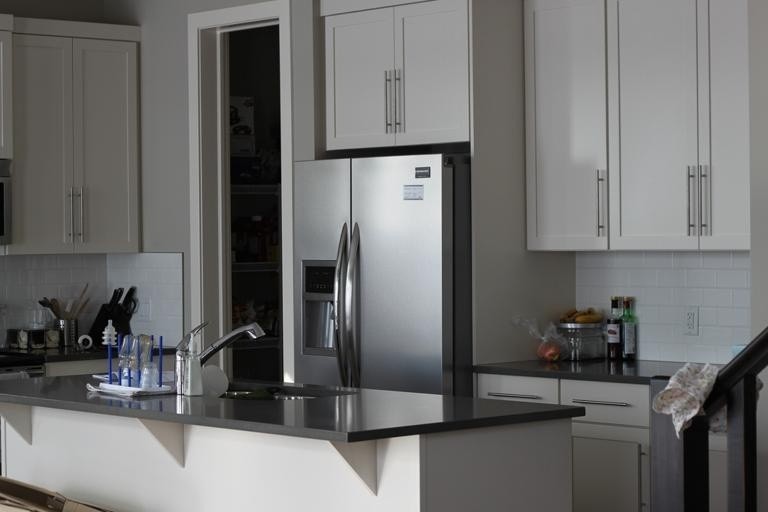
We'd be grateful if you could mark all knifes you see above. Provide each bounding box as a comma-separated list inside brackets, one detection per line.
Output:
[109, 287, 138, 309]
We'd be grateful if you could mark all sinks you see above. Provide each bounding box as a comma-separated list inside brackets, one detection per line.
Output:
[221, 391, 319, 400]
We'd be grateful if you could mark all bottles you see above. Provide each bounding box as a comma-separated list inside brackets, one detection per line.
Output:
[606, 361, 637, 376]
[607, 295, 624, 360]
[620, 296, 637, 365]
[118, 334, 153, 371]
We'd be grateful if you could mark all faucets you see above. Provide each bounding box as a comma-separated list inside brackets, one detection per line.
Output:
[176, 321, 266, 365]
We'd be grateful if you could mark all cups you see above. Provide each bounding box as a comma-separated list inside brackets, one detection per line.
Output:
[141, 363, 158, 392]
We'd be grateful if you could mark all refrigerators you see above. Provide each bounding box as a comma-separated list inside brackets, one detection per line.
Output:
[292, 152, 472, 393]
[283, 382, 474, 432]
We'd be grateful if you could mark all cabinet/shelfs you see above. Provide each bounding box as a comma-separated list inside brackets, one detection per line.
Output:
[232, 183, 282, 384]
[474, 373, 709, 512]
[525, 0, 610, 251]
[605, 0, 767, 251]
[321, 0, 471, 152]
[13, 33, 141, 256]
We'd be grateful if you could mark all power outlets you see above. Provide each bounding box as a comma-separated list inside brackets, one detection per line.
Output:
[682, 306, 699, 336]
